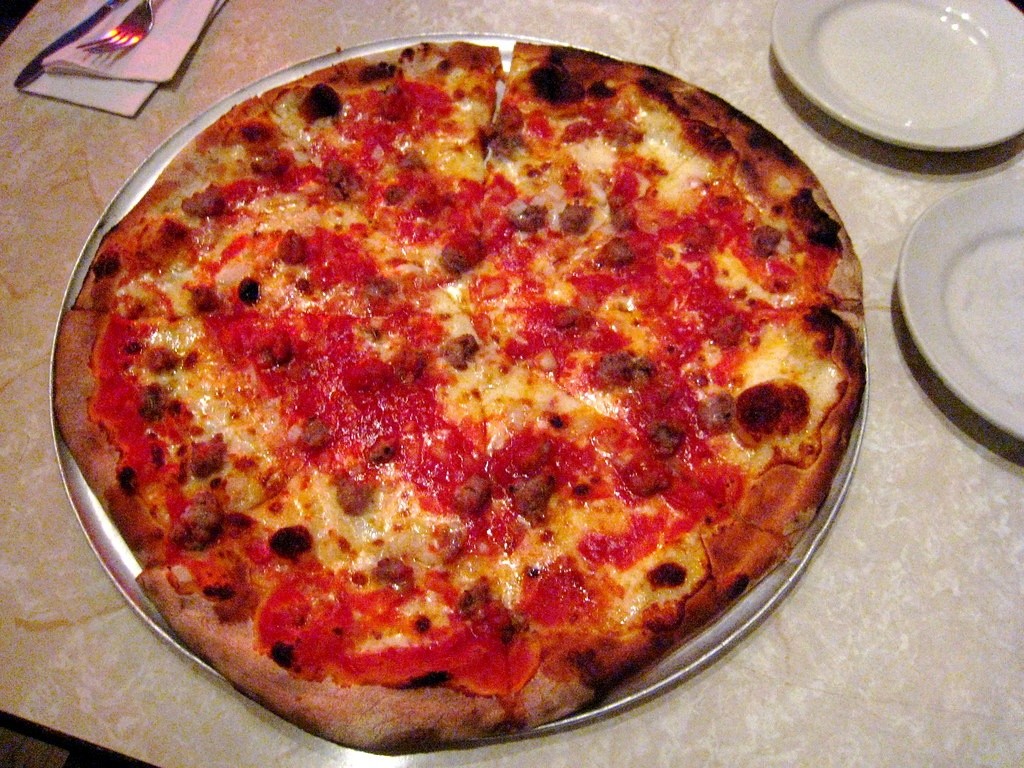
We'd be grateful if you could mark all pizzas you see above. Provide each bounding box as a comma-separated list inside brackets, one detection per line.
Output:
[53, 40, 867, 751]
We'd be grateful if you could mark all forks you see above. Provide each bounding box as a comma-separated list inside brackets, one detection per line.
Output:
[74, 1, 158, 57]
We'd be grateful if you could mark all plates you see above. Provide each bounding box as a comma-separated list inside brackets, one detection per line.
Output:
[899, 177, 1024, 444]
[49, 32, 868, 745]
[768, 2, 1024, 152]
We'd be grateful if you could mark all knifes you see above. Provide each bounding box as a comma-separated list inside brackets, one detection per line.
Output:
[11, 1, 125, 90]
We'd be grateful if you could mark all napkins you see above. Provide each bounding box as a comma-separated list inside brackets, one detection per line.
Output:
[17, 0, 226, 119]
